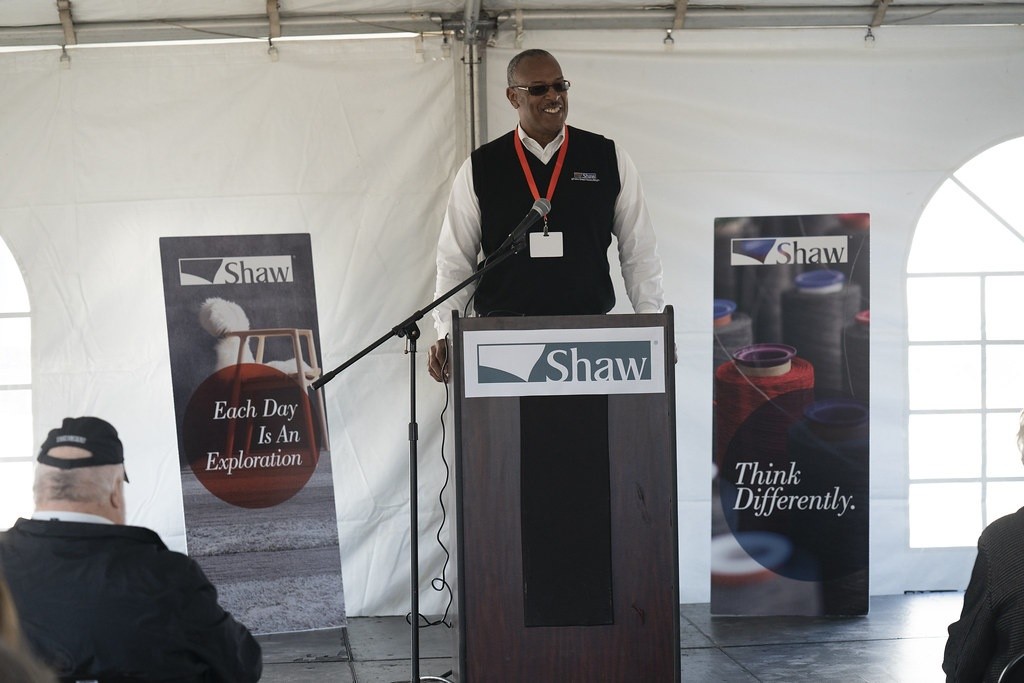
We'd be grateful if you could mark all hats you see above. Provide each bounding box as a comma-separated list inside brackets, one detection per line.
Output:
[37, 417, 129, 483]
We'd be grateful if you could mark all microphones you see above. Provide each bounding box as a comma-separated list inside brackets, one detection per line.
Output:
[496, 198, 551, 254]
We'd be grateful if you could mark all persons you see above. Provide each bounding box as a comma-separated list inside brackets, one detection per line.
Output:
[942, 411, 1024, 682]
[0, 416, 264, 683]
[424, 49, 678, 382]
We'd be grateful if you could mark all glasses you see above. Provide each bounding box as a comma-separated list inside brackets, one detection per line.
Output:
[512, 79, 570, 96]
